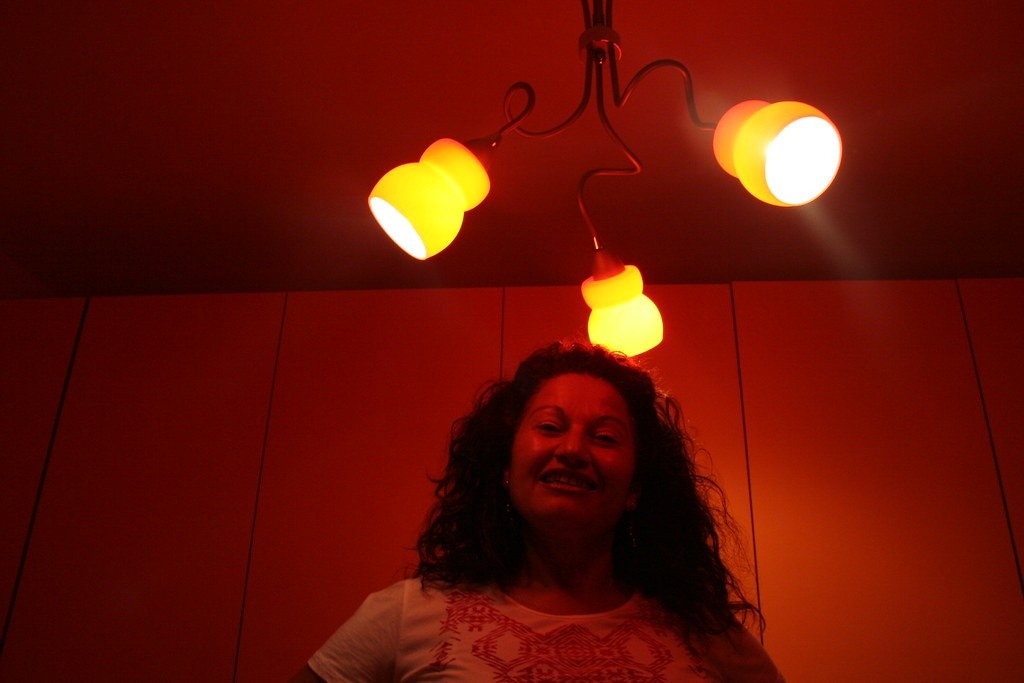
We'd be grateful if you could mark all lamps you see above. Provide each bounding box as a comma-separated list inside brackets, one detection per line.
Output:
[366, 0, 842, 359]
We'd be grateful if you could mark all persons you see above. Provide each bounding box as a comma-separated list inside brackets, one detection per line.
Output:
[289, 341, 785, 683]
